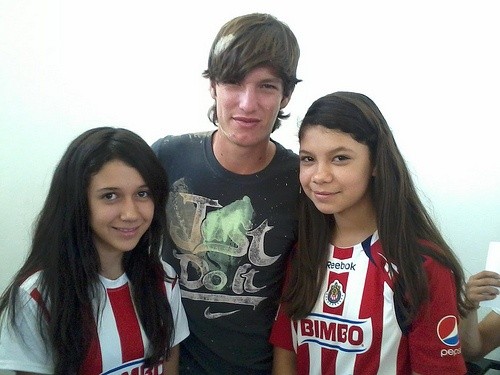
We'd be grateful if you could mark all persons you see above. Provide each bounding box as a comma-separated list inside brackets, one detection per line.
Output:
[268, 91, 475, 375]
[459, 268, 500, 375]
[0, 126, 190, 375]
[151, 13, 300, 375]
